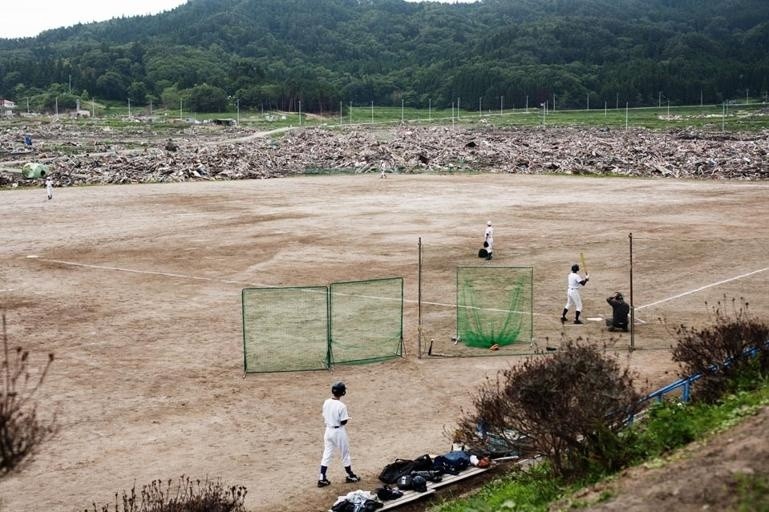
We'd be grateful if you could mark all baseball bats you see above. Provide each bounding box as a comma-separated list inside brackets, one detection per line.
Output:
[581, 252, 587, 275]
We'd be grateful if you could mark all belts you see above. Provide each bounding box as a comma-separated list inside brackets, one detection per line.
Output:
[335, 426, 339, 428]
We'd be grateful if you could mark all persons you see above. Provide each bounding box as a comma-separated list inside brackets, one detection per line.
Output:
[604, 290, 630, 331]
[483, 220, 495, 260]
[559, 265, 591, 324]
[317, 379, 362, 489]
[45, 176, 54, 201]
[379, 160, 387, 180]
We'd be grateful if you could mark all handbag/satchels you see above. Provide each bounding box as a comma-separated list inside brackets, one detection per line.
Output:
[378, 441, 494, 484]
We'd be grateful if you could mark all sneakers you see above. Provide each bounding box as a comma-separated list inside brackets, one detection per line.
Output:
[574, 320, 582, 324]
[561, 316, 567, 321]
[318, 479, 330, 487]
[345, 476, 360, 483]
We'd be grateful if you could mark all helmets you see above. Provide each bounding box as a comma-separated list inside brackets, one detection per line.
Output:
[614, 292, 623, 300]
[375, 474, 427, 499]
[332, 382, 346, 396]
[571, 264, 579, 272]
